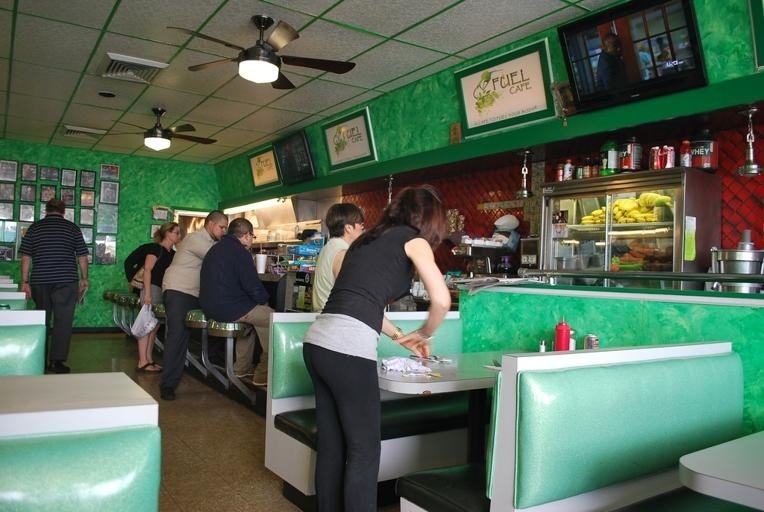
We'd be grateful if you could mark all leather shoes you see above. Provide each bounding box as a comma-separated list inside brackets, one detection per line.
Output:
[160, 384, 175, 401]
[47, 362, 70, 374]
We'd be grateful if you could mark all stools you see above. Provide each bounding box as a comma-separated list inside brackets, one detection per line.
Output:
[152, 304, 209, 377]
[128, 295, 190, 368]
[119, 294, 161, 353]
[207, 320, 259, 406]
[187, 309, 229, 390]
[104, 290, 129, 336]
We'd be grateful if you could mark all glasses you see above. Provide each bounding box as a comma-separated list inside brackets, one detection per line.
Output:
[243, 232, 256, 239]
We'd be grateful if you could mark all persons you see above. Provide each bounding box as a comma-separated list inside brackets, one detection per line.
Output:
[160, 211, 228, 401]
[639, 43, 654, 79]
[598, 35, 628, 89]
[136, 223, 180, 375]
[314, 203, 365, 313]
[20, 200, 88, 376]
[200, 220, 279, 387]
[303, 186, 451, 511]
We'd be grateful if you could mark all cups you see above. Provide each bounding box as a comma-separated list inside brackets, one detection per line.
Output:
[255, 254, 267, 275]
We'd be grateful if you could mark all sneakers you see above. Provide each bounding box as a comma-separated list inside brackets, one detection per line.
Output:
[234, 365, 256, 377]
[253, 375, 268, 386]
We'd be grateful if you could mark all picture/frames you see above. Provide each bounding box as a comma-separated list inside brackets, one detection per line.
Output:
[86, 248, 93, 265]
[80, 228, 94, 245]
[0, 159, 18, 182]
[0, 245, 14, 262]
[60, 188, 76, 207]
[0, 203, 14, 220]
[39, 204, 48, 220]
[79, 208, 95, 226]
[40, 166, 60, 182]
[152, 206, 169, 221]
[98, 181, 120, 205]
[14, 223, 34, 261]
[40, 184, 57, 203]
[81, 189, 95, 208]
[319, 104, 378, 171]
[171, 207, 210, 253]
[19, 184, 36, 202]
[452, 36, 560, 142]
[249, 145, 282, 189]
[100, 164, 120, 180]
[150, 224, 162, 239]
[79, 170, 96, 189]
[21, 162, 37, 182]
[64, 208, 75, 224]
[20, 204, 35, 222]
[1, 183, 15, 202]
[61, 168, 77, 187]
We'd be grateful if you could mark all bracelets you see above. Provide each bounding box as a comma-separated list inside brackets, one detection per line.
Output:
[415, 328, 435, 342]
[390, 327, 403, 340]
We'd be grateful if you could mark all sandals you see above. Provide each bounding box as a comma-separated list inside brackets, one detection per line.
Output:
[136, 363, 161, 375]
[150, 361, 163, 372]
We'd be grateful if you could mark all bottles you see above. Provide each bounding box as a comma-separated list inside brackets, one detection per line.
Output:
[584, 334, 598, 349]
[563, 159, 573, 181]
[591, 157, 599, 177]
[575, 162, 582, 179]
[555, 322, 570, 351]
[582, 158, 591, 179]
[539, 340, 545, 353]
[570, 330, 576, 350]
[680, 140, 692, 167]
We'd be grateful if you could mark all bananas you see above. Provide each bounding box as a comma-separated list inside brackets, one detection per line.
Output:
[581, 191, 673, 225]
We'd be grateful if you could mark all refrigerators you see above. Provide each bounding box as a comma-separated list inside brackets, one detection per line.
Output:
[537, 168, 722, 291]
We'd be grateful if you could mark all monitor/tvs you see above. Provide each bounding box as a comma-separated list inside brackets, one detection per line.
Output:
[557, 0, 711, 116]
[272, 127, 316, 187]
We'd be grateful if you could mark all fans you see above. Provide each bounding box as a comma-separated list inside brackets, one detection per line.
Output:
[105, 106, 218, 151]
[164, 13, 356, 90]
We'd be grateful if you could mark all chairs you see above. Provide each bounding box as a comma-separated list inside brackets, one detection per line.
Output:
[0, 310, 47, 374]
[0, 291, 28, 309]
[0, 372, 161, 512]
[0, 284, 19, 292]
[394, 341, 745, 512]
[0, 275, 10, 279]
[265, 308, 472, 511]
[1, 279, 14, 283]
